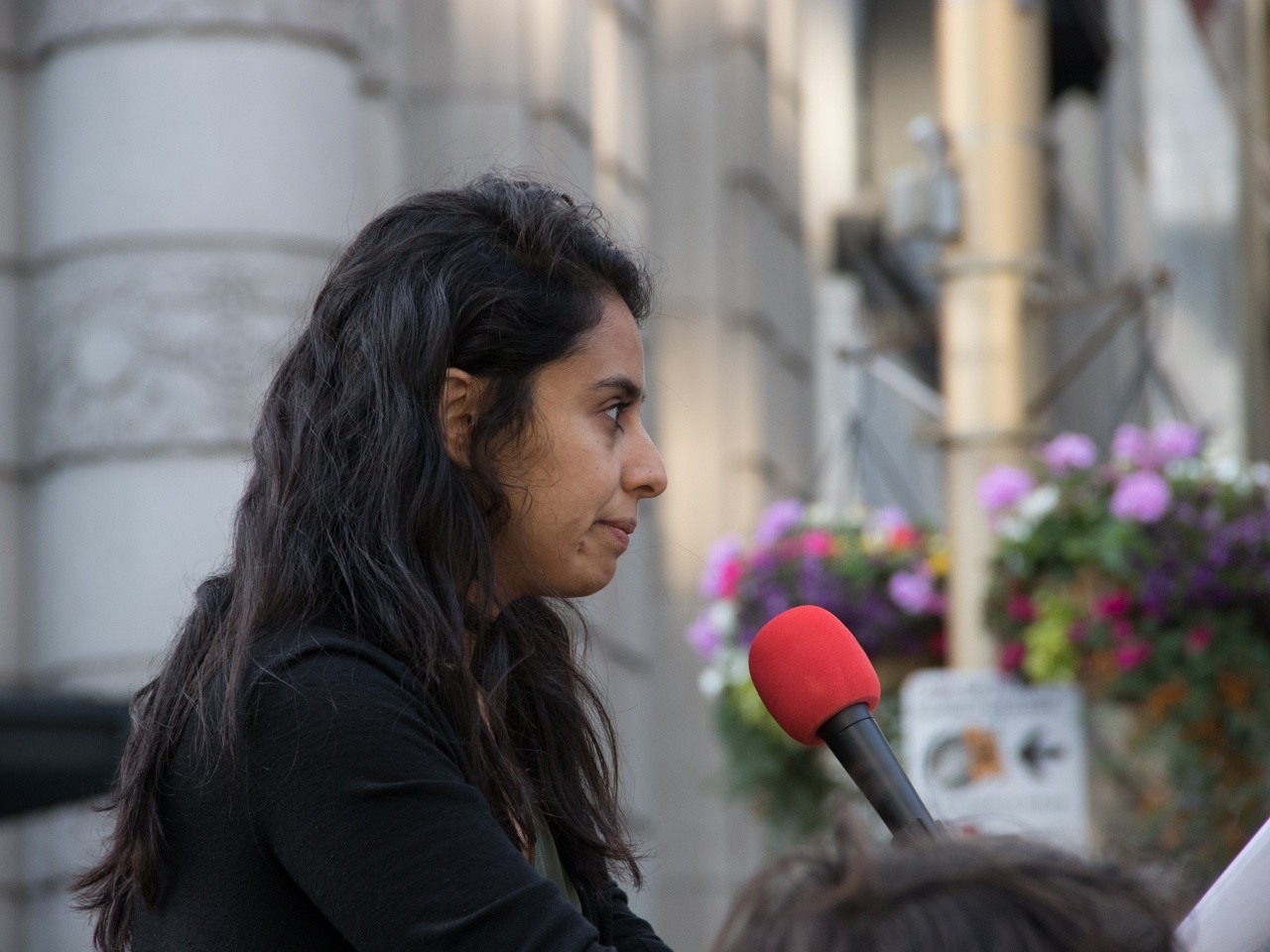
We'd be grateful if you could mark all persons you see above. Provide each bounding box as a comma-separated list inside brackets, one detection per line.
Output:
[711, 832, 1184, 952]
[66, 167, 677, 952]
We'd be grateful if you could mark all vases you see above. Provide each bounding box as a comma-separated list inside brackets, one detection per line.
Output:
[1085, 676, 1270, 863]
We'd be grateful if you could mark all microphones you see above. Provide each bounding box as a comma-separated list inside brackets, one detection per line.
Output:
[748, 605, 954, 855]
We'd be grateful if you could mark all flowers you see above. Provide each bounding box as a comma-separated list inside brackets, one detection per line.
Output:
[687, 493, 950, 846]
[987, 415, 1270, 753]
